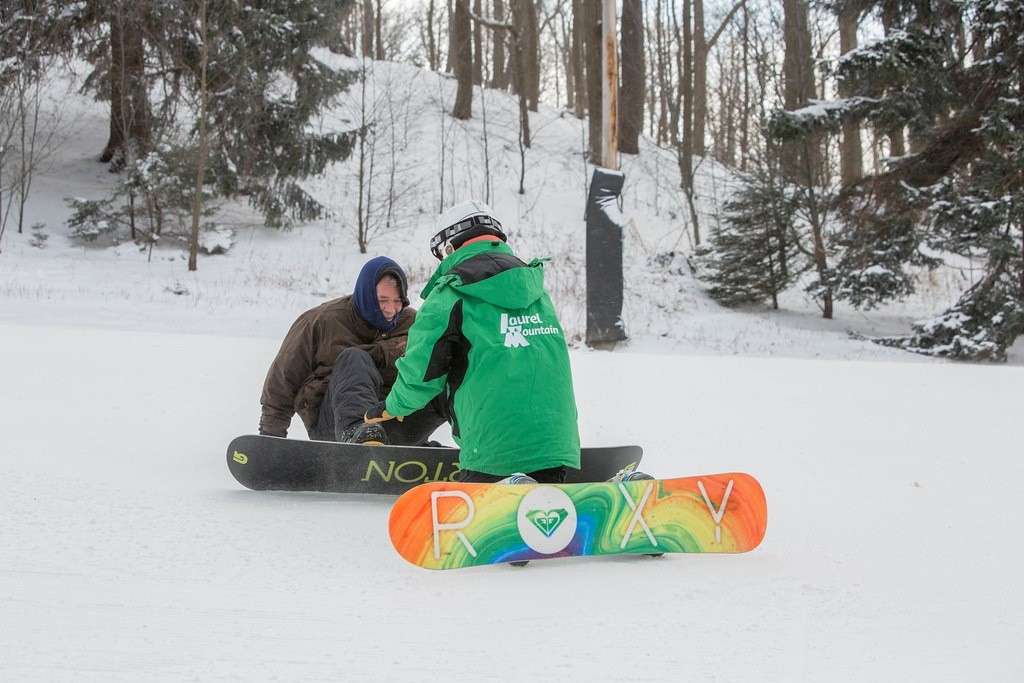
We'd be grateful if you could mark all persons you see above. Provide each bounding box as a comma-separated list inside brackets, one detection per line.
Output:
[363, 199, 667, 566]
[258, 255, 448, 447]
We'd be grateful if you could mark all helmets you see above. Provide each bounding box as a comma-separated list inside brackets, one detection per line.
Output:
[429, 201, 504, 260]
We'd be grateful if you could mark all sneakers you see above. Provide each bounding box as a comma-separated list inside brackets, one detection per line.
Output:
[350, 424, 387, 445]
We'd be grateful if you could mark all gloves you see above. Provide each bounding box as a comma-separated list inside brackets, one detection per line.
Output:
[363, 400, 403, 425]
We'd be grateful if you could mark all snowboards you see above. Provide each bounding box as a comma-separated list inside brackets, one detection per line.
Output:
[390, 472, 768, 570]
[225, 434, 644, 496]
